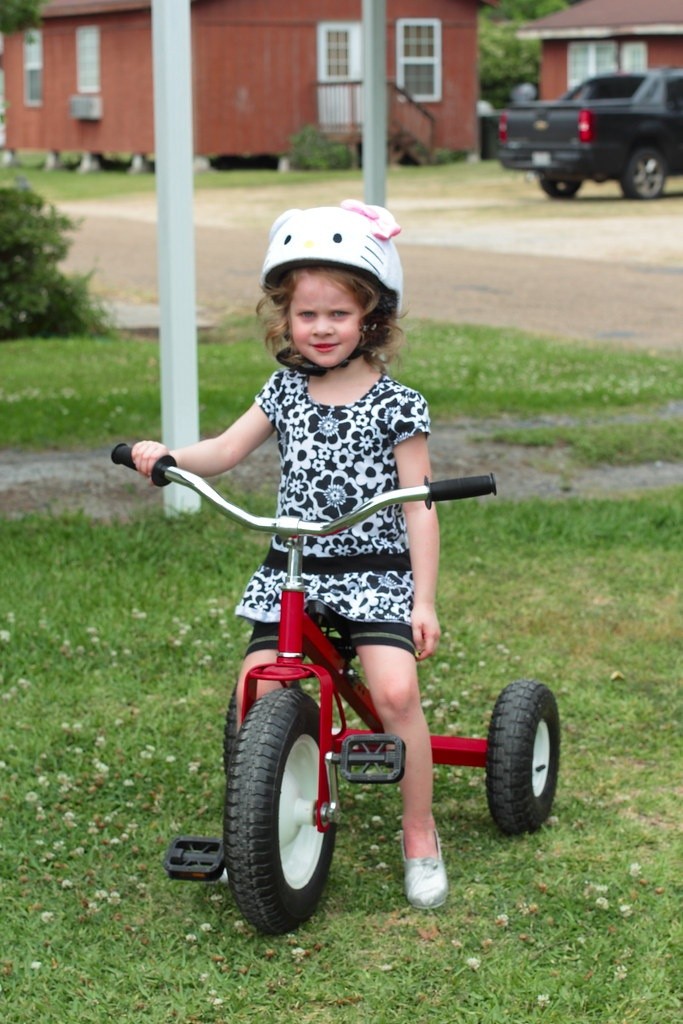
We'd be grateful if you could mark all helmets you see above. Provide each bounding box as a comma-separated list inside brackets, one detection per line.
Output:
[260, 199, 403, 322]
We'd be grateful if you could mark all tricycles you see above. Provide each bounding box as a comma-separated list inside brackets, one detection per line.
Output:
[108, 441, 559, 938]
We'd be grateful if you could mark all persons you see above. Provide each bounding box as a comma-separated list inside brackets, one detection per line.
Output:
[132, 200, 446, 907]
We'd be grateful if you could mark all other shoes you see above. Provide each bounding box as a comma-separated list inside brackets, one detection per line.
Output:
[401, 829, 447, 910]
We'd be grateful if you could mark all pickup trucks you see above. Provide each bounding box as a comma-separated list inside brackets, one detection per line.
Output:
[494, 70, 683, 202]
